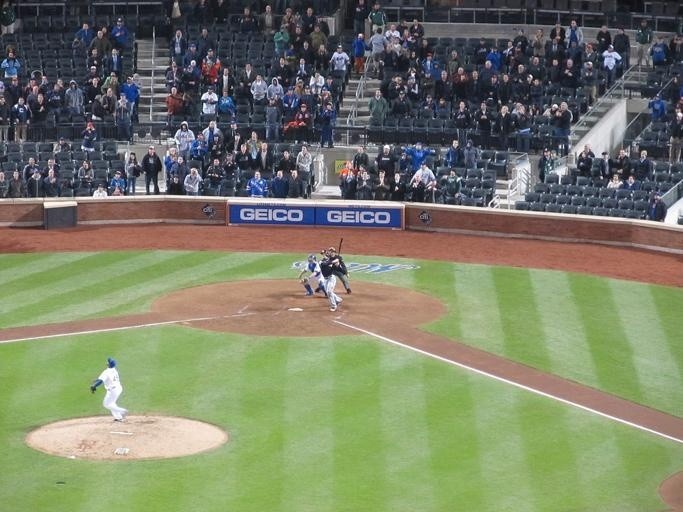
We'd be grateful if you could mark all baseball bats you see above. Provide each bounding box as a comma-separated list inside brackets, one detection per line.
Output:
[338, 238, 343, 256]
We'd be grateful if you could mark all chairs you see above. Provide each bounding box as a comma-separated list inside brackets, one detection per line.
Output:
[0, 0, 682, 226]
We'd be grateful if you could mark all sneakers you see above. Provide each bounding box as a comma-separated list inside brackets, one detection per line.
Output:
[330, 306, 337, 311]
[346, 289, 351, 294]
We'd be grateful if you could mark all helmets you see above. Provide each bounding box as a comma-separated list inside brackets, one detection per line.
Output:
[328, 247, 336, 253]
[320, 250, 326, 255]
[308, 255, 315, 262]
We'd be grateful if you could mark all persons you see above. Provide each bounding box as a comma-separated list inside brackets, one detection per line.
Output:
[1, 0, 141, 198]
[340, 138, 480, 202]
[299, 253, 326, 298]
[164, 1, 349, 199]
[142, 146, 162, 195]
[367, 3, 629, 153]
[313, 246, 351, 295]
[90, 359, 128, 423]
[351, 33, 370, 75]
[350, 1, 370, 35]
[538, 144, 651, 189]
[643, 192, 667, 222]
[636, 19, 683, 163]
[319, 249, 343, 313]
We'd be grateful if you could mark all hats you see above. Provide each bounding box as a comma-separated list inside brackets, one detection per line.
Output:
[384, 145, 389, 149]
[108, 357, 115, 368]
